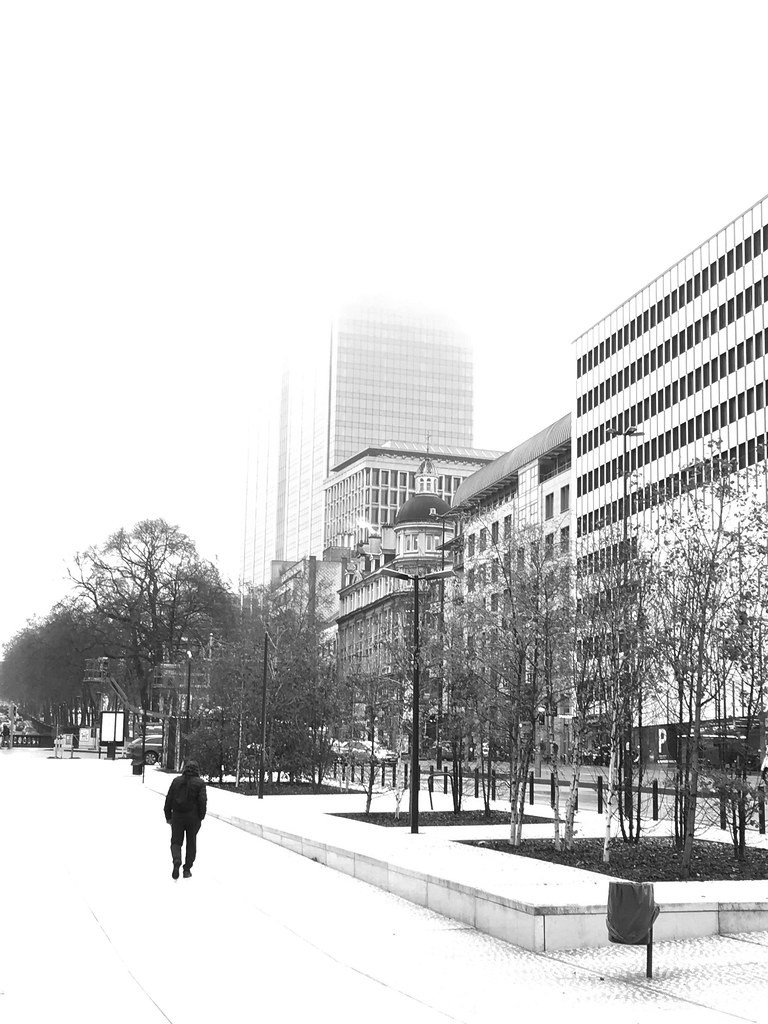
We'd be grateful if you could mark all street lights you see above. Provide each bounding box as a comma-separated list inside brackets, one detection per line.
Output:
[379, 567, 456, 837]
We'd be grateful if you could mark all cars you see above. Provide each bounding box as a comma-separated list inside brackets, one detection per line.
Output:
[127, 735, 165, 765]
[341, 740, 399, 766]
[425, 739, 506, 762]
[331, 739, 355, 763]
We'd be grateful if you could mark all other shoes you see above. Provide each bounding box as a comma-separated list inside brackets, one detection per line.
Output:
[183, 870, 192, 877]
[172, 859, 181, 878]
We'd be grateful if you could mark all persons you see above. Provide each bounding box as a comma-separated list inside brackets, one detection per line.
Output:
[1, 724, 9, 747]
[164, 760, 208, 879]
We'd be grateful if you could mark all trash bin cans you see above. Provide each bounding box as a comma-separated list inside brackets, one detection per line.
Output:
[131, 758, 145, 775]
[606, 880, 658, 945]
